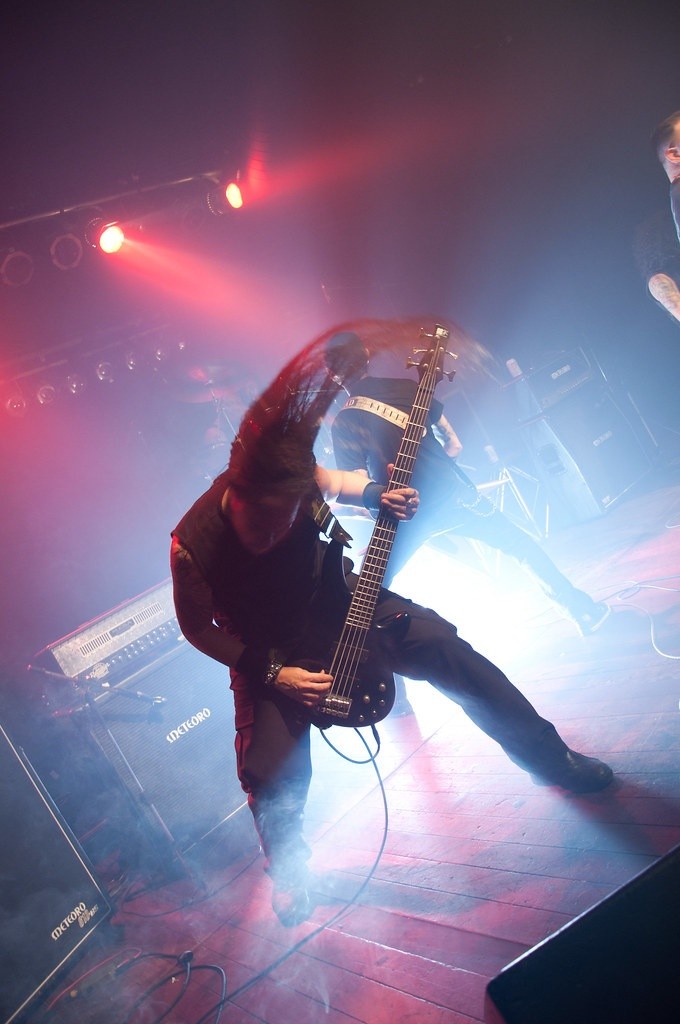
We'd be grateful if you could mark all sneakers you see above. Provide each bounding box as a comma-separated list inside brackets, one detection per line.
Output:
[269, 865, 316, 922]
[581, 601, 610, 630]
[529, 747, 615, 794]
[388, 698, 412, 717]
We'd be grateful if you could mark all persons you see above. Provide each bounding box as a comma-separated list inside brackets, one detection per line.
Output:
[652, 109, 679, 245]
[320, 331, 610, 635]
[399, 313, 591, 545]
[167, 318, 613, 927]
[631, 204, 680, 325]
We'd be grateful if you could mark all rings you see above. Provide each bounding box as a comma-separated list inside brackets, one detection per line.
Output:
[407, 497, 412, 507]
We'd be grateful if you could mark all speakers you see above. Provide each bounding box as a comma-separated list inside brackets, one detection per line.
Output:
[481, 839, 680, 1024]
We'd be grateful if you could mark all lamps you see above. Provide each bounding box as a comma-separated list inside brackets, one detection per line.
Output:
[172, 194, 207, 236]
[79, 214, 126, 254]
[47, 233, 85, 271]
[1, 249, 36, 289]
[205, 180, 244, 220]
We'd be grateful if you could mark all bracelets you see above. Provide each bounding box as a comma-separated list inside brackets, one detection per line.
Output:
[263, 658, 283, 690]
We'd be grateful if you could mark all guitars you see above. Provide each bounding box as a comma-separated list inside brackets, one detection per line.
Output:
[263, 322, 458, 729]
